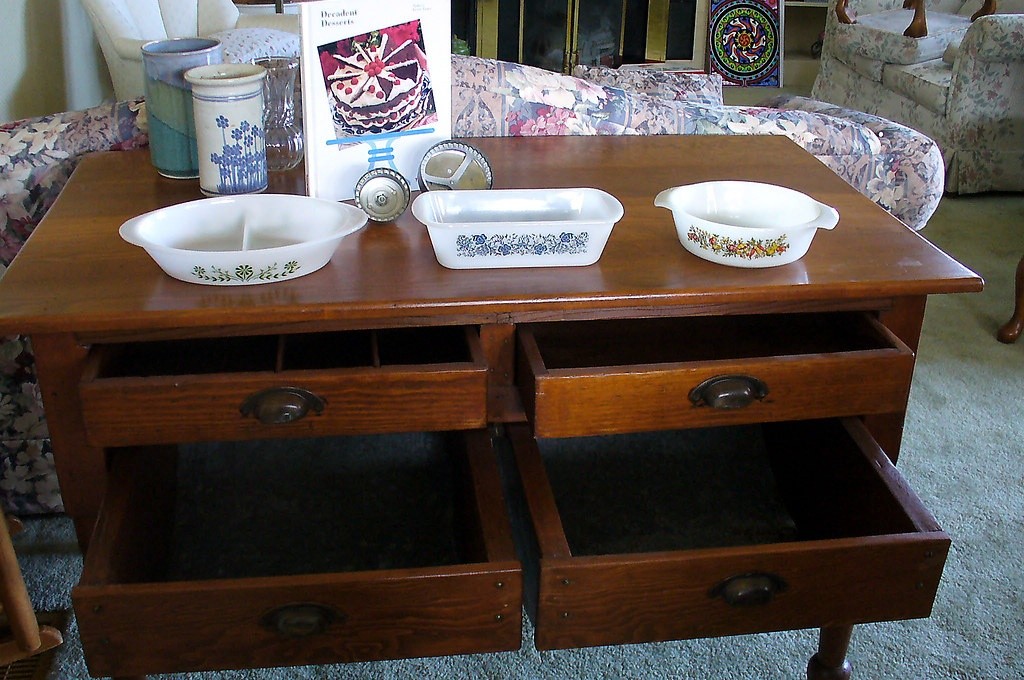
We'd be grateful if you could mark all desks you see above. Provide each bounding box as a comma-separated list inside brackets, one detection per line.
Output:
[1, 135, 986, 679]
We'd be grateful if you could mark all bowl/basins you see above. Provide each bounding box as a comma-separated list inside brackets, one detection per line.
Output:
[653, 179, 840, 267]
[118, 193, 368, 287]
[411, 187, 624, 269]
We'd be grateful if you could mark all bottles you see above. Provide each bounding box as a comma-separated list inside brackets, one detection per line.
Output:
[245, 55, 304, 171]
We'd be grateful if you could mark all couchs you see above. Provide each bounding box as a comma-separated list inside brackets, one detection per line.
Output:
[810, 0, 1024, 198]
[0, 28, 946, 516]
[76, 0, 308, 106]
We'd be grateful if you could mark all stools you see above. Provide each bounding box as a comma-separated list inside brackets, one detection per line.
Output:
[833, 0, 998, 64]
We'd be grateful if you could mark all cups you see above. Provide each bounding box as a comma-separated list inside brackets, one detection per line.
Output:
[184, 63, 269, 197]
[140, 36, 223, 180]
